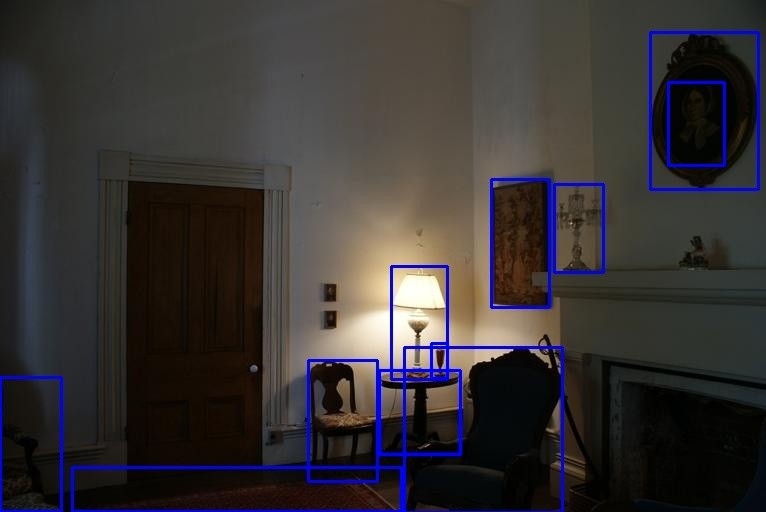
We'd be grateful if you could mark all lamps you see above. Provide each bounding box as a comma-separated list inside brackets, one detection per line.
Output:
[405, 347, 562, 512]
[0, 378, 61, 512]
[309, 361, 378, 482]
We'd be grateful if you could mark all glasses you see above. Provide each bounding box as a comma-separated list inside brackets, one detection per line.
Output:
[391, 266, 447, 380]
[555, 184, 604, 272]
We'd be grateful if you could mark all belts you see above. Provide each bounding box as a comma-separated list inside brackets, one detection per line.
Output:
[432, 344, 447, 378]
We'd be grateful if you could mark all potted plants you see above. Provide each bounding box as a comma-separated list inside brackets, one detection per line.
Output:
[650, 32, 758, 190]
[491, 180, 549, 308]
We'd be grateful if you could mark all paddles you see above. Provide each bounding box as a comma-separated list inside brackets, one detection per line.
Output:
[73, 468, 402, 512]
